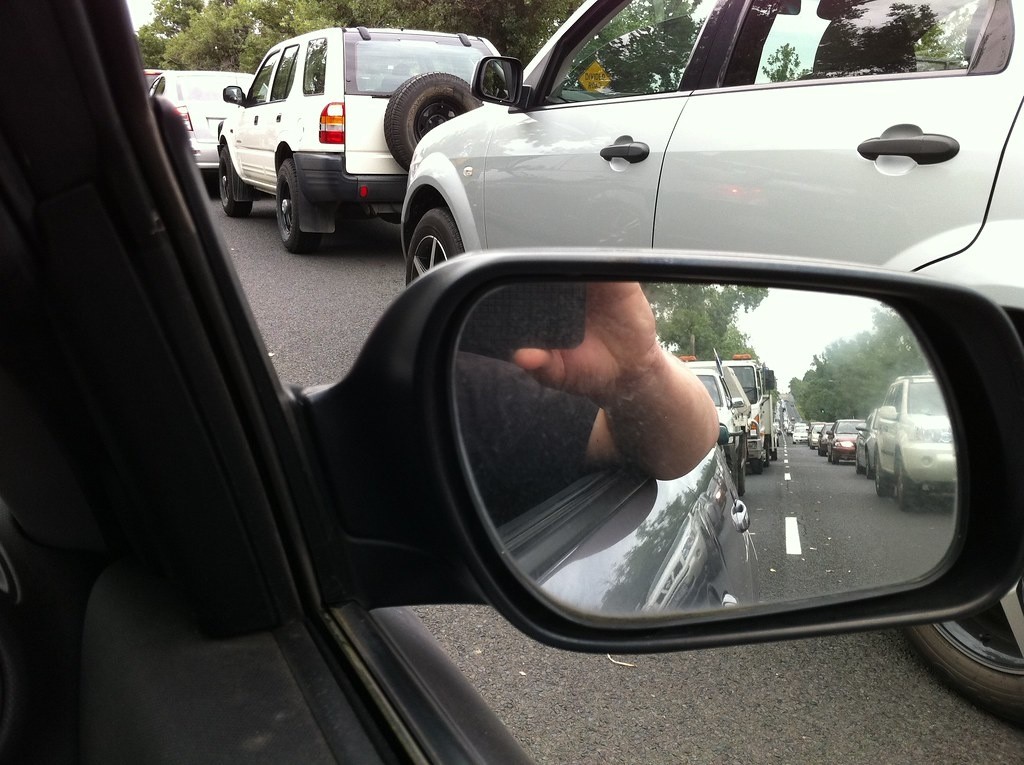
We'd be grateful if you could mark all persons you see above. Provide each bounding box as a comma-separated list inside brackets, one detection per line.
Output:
[454, 281, 719, 528]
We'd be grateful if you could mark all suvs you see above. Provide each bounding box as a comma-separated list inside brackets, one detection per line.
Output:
[873, 375, 958, 512]
[217, 27, 503, 254]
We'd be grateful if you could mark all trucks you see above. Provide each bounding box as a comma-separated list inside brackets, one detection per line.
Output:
[679, 354, 782, 475]
[678, 369, 752, 497]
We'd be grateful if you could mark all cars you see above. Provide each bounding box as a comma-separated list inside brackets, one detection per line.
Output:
[144, 69, 165, 87]
[826, 419, 866, 465]
[817, 423, 835, 456]
[855, 408, 880, 480]
[148, 70, 277, 169]
[402, 1, 1024, 725]
[786, 422, 826, 450]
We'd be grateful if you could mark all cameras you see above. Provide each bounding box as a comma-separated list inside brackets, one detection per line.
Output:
[463, 282, 586, 350]
